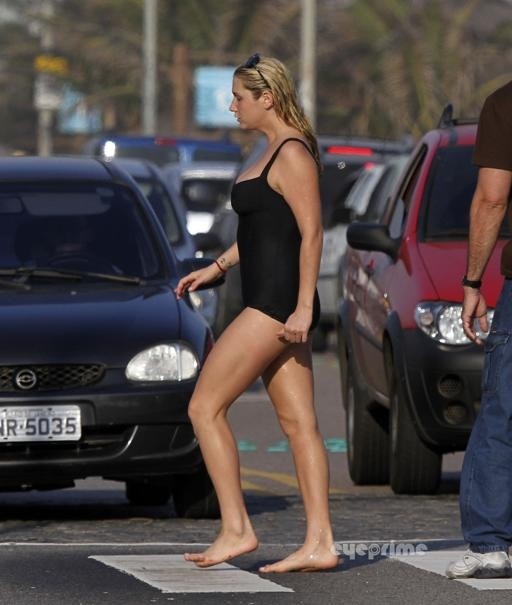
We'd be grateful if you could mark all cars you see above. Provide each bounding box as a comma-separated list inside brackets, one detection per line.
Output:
[0, 156, 226, 514]
[85, 133, 415, 344]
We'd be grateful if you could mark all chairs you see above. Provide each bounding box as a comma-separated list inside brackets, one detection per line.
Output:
[444, 550, 512, 580]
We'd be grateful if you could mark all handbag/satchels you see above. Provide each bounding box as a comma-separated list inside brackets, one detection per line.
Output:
[462, 276, 481, 288]
[215, 260, 227, 275]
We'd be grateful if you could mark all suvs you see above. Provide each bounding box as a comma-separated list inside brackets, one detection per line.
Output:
[335, 107, 508, 496]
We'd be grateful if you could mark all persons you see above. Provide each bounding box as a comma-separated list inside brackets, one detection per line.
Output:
[446, 82, 511, 579]
[174, 53, 338, 574]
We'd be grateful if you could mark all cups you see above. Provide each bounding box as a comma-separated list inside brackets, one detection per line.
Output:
[244, 51, 271, 89]
